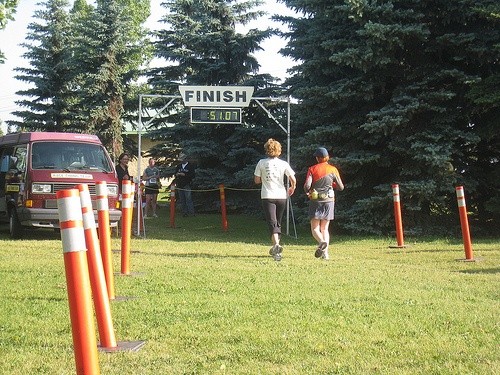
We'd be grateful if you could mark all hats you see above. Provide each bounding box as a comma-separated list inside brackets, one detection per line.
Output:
[313, 148, 329, 158]
[178, 154, 186, 161]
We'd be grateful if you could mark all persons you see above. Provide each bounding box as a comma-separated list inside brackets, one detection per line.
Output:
[142, 158, 161, 218]
[304, 147, 344, 260]
[174, 154, 196, 217]
[112, 153, 130, 233]
[254, 138, 296, 261]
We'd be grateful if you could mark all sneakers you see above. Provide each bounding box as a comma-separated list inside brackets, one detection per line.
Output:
[183, 212, 195, 217]
[143, 214, 160, 219]
[269, 245, 283, 261]
[313, 242, 329, 260]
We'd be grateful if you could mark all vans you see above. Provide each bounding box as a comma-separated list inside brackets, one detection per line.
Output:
[0, 132, 122, 238]
